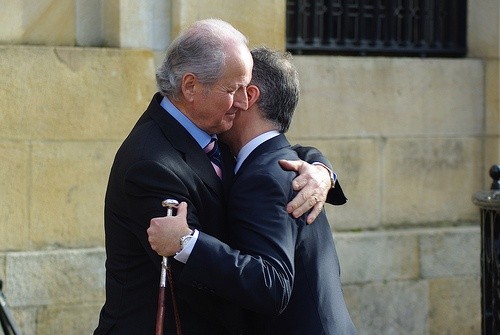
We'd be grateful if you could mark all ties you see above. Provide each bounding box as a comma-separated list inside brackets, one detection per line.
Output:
[204, 140, 231, 188]
